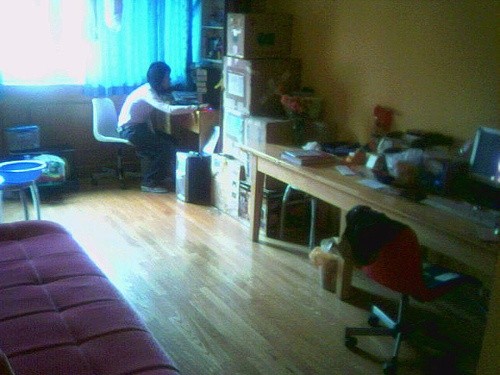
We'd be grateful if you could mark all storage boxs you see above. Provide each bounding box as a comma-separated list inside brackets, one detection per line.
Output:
[239, 181, 327, 237]
[222, 57, 302, 117]
[244, 117, 293, 147]
[246, 156, 297, 191]
[210, 152, 246, 219]
[5, 125, 41, 155]
[226, 11, 290, 59]
[223, 108, 244, 142]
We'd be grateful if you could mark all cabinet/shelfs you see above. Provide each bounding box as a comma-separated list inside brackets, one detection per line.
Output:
[197, 1, 225, 101]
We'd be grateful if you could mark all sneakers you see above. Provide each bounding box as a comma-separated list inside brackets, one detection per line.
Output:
[140, 185, 168, 192]
[159, 176, 174, 183]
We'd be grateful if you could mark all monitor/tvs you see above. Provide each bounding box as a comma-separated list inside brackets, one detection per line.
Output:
[468, 125, 500, 212]
[207, 69, 222, 108]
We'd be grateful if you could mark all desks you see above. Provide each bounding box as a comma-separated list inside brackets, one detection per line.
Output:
[241, 143, 500, 374]
[152, 92, 223, 154]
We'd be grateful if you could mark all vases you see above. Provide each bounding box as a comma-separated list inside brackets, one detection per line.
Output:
[291, 120, 307, 146]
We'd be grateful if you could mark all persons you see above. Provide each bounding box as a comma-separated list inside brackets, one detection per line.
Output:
[117, 62, 213, 193]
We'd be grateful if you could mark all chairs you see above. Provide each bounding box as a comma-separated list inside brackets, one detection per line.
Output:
[91, 99, 142, 188]
[345, 205, 465, 375]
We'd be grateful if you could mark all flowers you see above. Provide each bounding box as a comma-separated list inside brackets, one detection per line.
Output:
[272, 82, 316, 120]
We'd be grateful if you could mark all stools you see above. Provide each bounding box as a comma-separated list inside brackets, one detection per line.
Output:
[0, 181, 41, 222]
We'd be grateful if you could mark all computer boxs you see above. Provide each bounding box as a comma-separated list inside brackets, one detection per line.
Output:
[175, 151, 212, 203]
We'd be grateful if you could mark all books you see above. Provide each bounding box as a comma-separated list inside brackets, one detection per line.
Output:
[285, 150, 322, 158]
[280, 149, 335, 166]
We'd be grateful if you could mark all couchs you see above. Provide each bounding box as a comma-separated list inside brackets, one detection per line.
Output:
[1, 221, 179, 375]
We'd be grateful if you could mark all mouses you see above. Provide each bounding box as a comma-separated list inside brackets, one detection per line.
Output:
[208, 106, 216, 112]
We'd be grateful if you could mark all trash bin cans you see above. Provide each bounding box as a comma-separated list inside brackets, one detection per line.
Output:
[314, 236, 353, 292]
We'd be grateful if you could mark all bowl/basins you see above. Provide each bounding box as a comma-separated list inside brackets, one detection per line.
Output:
[0, 160, 47, 184]
